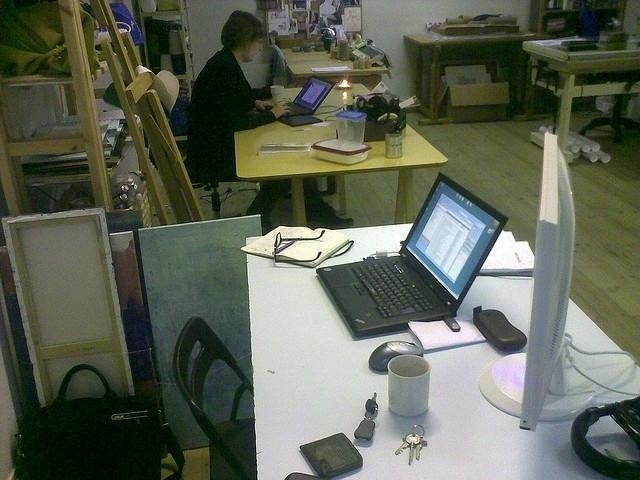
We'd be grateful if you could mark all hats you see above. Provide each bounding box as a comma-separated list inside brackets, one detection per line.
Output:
[220, 10, 266, 44]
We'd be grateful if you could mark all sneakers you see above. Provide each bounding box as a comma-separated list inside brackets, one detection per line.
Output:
[307, 209, 355, 228]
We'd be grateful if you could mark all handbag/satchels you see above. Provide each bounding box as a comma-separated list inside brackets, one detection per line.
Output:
[16, 361, 162, 480]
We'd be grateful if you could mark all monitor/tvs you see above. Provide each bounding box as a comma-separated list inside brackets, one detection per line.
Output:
[480, 131, 598, 430]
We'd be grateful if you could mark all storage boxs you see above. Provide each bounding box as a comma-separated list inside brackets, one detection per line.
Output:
[435, 75, 510, 123]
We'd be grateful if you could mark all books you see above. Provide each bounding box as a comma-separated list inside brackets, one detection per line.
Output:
[21, 99, 143, 177]
[256, 142, 311, 157]
[543, 0, 625, 32]
[240, 225, 350, 268]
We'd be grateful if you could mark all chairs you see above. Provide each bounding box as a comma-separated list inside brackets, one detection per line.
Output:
[573, 8, 640, 142]
[173, 317, 256, 480]
[270, 44, 287, 87]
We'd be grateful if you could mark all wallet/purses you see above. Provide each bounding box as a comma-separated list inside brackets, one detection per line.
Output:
[299, 432, 363, 480]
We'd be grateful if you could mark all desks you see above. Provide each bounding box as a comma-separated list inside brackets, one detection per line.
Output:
[521, 31, 639, 153]
[234, 83, 448, 229]
[402, 31, 537, 116]
[281, 47, 387, 92]
[244, 219, 639, 480]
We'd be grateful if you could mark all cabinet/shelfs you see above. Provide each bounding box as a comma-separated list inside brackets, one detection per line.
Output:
[1, 0, 143, 212]
[133, 0, 196, 103]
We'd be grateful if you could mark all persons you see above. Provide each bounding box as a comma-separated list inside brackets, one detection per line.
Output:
[184, 9, 356, 229]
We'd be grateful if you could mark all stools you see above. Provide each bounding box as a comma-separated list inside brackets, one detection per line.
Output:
[185, 159, 241, 221]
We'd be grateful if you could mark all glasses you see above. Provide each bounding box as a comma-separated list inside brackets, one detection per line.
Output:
[272, 228, 326, 265]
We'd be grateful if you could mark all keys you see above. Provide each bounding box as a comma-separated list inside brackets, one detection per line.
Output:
[394, 432, 424, 465]
[365, 392, 379, 415]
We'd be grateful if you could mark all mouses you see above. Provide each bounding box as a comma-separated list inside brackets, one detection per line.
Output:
[368, 340, 424, 372]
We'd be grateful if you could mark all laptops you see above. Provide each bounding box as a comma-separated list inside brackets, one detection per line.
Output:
[271, 77, 336, 117]
[316, 172, 509, 340]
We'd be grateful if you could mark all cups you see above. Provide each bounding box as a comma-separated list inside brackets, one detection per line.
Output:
[384, 133, 403, 159]
[335, 110, 367, 148]
[269, 84, 285, 105]
[386, 353, 431, 418]
